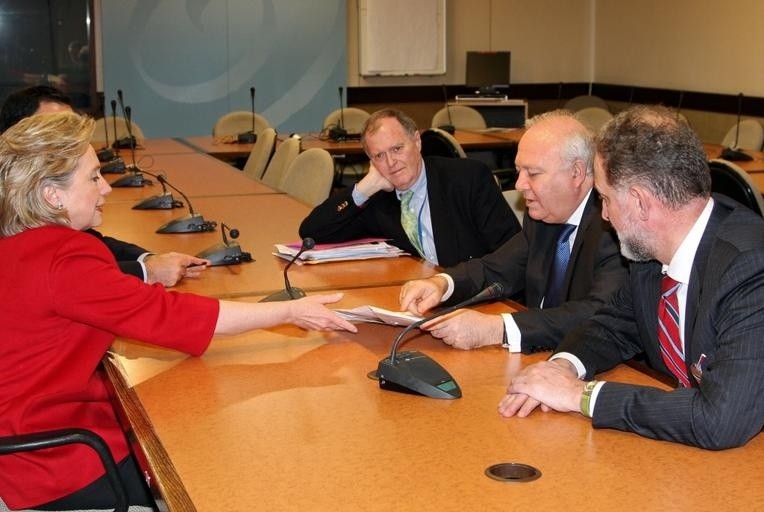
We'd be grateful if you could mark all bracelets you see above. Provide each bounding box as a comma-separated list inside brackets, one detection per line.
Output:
[580, 379, 597, 417]
[501, 322, 509, 349]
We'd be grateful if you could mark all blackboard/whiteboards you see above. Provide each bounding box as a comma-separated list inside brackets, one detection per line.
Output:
[359, 0, 448, 77]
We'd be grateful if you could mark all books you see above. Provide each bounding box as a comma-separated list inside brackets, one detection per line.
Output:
[271, 238, 412, 267]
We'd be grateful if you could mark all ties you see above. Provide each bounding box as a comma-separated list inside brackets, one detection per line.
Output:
[655, 274, 693, 388]
[399, 191, 426, 260]
[546, 225, 576, 309]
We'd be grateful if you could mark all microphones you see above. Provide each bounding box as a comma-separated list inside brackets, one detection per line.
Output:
[198, 222, 250, 268]
[256, 235, 315, 303]
[131, 166, 175, 210]
[113, 88, 138, 150]
[237, 86, 257, 145]
[155, 174, 205, 235]
[722, 92, 754, 166]
[437, 83, 456, 133]
[328, 86, 350, 140]
[111, 107, 142, 188]
[97, 91, 113, 161]
[377, 280, 504, 400]
[100, 100, 124, 175]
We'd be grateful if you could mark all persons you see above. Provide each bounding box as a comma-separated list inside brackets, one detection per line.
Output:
[1, 112, 357, 511]
[1, 84, 212, 287]
[399, 109, 629, 354]
[298, 108, 522, 268]
[497, 102, 764, 451]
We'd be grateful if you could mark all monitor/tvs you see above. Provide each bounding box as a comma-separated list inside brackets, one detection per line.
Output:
[464, 50, 512, 95]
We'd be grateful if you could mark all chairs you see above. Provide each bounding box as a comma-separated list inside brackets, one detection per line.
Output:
[0, 104, 764, 511]
